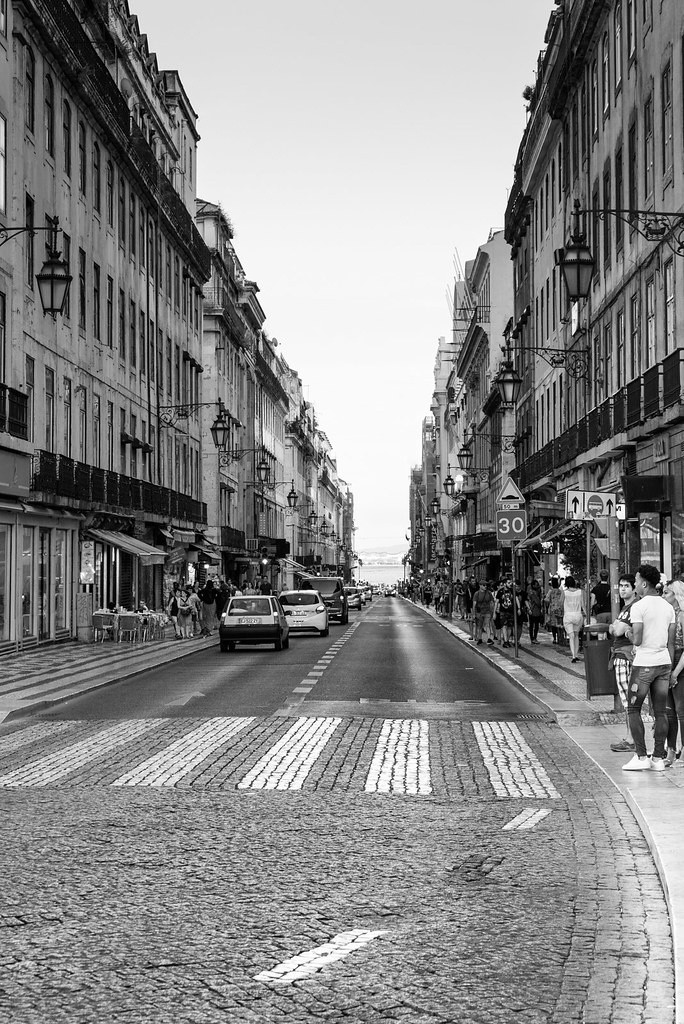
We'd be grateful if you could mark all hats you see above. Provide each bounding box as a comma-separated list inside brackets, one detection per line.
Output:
[480, 580, 489, 584]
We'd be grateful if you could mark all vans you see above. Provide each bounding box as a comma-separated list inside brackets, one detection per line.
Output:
[297, 576, 352, 625]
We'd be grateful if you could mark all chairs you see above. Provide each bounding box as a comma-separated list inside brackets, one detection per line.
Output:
[92, 613, 164, 643]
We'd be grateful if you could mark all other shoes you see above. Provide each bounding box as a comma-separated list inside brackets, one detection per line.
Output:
[477, 636, 561, 649]
[572, 657, 580, 663]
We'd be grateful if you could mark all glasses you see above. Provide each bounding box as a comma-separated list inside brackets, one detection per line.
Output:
[482, 585, 487, 586]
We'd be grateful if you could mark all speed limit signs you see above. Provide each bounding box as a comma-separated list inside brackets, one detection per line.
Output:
[496, 509, 527, 541]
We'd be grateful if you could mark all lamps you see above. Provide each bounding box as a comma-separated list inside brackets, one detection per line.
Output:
[443, 476, 465, 500]
[219, 447, 270, 482]
[497, 345, 591, 404]
[563, 198, 684, 300]
[331, 524, 337, 541]
[424, 513, 432, 527]
[419, 525, 425, 536]
[248, 479, 299, 508]
[300, 503, 318, 524]
[457, 432, 516, 470]
[320, 514, 328, 534]
[159, 399, 231, 450]
[337, 536, 347, 550]
[1, 216, 72, 321]
[430, 488, 441, 514]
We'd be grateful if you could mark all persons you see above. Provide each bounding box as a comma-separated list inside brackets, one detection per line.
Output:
[169, 575, 272, 639]
[134, 600, 148, 625]
[662, 580, 684, 768]
[610, 574, 638, 752]
[405, 577, 561, 648]
[591, 569, 612, 624]
[622, 564, 676, 772]
[560, 576, 586, 663]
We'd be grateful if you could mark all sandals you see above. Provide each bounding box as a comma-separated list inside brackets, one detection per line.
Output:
[664, 758, 671, 768]
[672, 758, 684, 768]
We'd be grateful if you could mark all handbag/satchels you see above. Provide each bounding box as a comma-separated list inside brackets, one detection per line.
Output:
[593, 604, 602, 613]
[477, 607, 481, 613]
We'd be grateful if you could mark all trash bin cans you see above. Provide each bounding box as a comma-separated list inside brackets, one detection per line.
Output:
[583, 624, 618, 696]
[444, 593, 453, 613]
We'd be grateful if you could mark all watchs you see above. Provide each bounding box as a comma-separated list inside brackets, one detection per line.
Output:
[625, 630, 631, 638]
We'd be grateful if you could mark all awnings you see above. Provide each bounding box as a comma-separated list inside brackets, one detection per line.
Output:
[88, 528, 168, 565]
[460, 557, 490, 570]
[160, 529, 221, 565]
[515, 520, 582, 565]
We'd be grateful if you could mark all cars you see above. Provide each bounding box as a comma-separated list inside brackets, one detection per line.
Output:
[219, 594, 293, 653]
[357, 584, 397, 602]
[276, 589, 330, 637]
[342, 586, 363, 611]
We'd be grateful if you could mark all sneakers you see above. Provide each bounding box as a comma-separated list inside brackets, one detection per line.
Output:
[650, 755, 665, 771]
[610, 740, 636, 752]
[621, 753, 651, 771]
